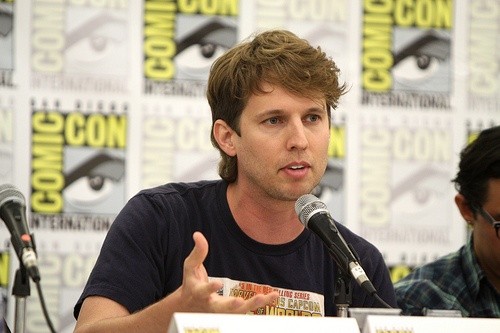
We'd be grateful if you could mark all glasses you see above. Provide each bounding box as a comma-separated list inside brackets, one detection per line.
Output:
[473, 204, 500, 240]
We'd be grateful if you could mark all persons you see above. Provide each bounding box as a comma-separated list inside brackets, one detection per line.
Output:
[72, 29, 399, 332]
[394, 125, 500, 319]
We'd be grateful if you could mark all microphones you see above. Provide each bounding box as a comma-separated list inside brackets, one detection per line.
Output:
[1, 185, 41, 281]
[295, 195, 376, 296]
